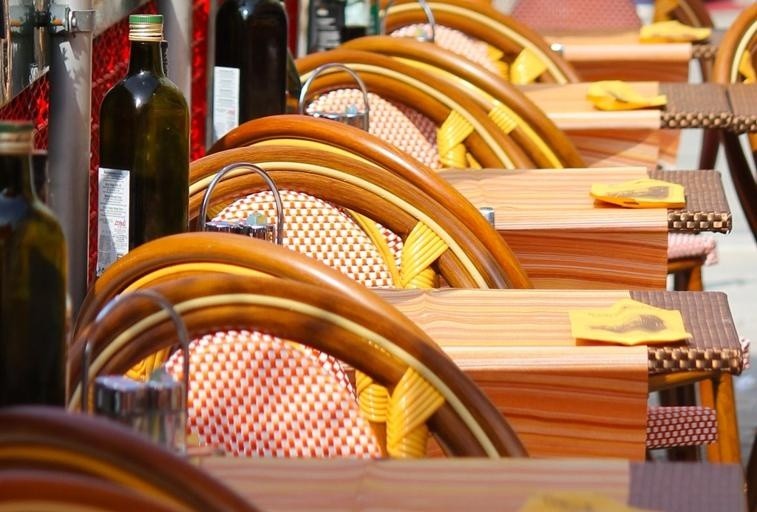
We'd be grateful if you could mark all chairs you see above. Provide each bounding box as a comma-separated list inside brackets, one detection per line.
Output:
[717, 2, 756, 240]
[290, 35, 589, 175]
[0, 404, 256, 512]
[68, 231, 717, 462]
[377, 1, 582, 82]
[186, 115, 718, 293]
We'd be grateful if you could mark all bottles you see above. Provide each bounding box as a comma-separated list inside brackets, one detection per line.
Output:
[0, 119, 68, 408]
[206, 0, 288, 158]
[97, 14, 190, 283]
